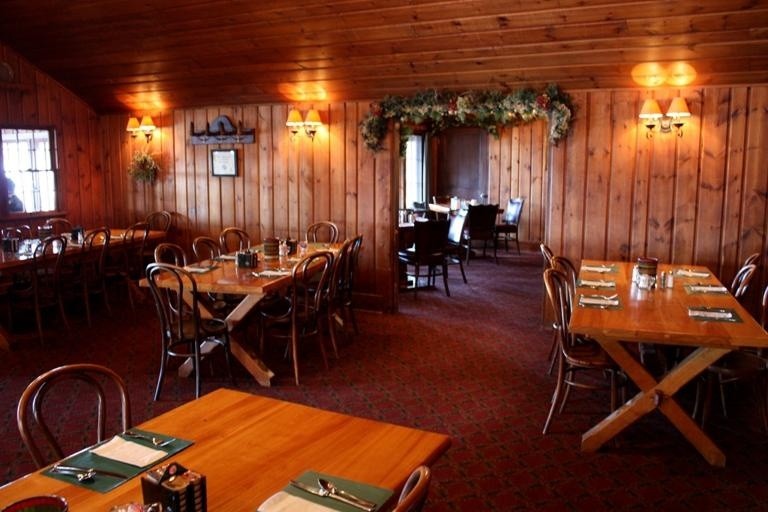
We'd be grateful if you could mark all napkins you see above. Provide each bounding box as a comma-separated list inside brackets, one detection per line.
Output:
[257, 491, 332, 510]
[89, 437, 165, 467]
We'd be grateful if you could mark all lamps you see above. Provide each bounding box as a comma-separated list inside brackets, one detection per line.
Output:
[283, 107, 322, 141]
[124, 116, 158, 146]
[638, 93, 689, 141]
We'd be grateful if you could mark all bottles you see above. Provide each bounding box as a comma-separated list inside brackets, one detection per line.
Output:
[660, 271, 666, 288]
[667, 270, 673, 287]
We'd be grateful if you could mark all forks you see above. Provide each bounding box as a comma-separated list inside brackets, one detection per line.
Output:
[586, 293, 618, 300]
[123, 431, 177, 446]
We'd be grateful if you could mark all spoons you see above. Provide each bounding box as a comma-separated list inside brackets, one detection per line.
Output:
[582, 303, 610, 309]
[50, 467, 97, 482]
[317, 477, 377, 507]
[251, 271, 277, 278]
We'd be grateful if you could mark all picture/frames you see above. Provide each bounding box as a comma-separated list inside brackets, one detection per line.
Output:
[210, 147, 238, 177]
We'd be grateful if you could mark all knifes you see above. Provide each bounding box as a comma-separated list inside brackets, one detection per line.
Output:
[55, 462, 128, 479]
[689, 307, 725, 313]
[289, 477, 376, 511]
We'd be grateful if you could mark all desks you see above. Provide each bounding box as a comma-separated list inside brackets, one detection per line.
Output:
[2, 389, 453, 511]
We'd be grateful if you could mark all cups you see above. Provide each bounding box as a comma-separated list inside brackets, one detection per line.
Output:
[0, 494, 70, 512]
[636, 274, 656, 290]
[263, 237, 298, 261]
[60, 233, 72, 247]
[109, 500, 144, 512]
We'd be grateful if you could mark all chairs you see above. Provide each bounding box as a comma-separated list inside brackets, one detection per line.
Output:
[3, 208, 173, 342]
[538, 243, 768, 469]
[16, 363, 127, 467]
[140, 222, 361, 390]
[394, 195, 525, 297]
[393, 466, 430, 511]
